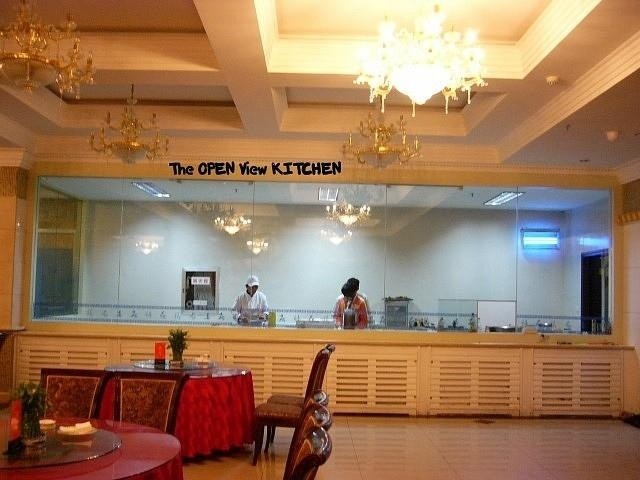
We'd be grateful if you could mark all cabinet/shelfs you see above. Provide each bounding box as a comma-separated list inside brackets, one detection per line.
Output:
[384, 300, 409, 328]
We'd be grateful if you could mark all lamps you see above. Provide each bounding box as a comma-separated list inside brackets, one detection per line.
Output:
[211, 203, 253, 237]
[321, 183, 373, 231]
[244, 233, 268, 258]
[338, 102, 418, 171]
[342, 4, 492, 121]
[92, 81, 169, 165]
[0, 0, 99, 100]
[131, 234, 164, 256]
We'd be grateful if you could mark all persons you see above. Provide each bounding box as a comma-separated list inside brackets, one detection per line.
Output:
[230, 275, 269, 327]
[331, 283, 369, 329]
[346, 277, 373, 328]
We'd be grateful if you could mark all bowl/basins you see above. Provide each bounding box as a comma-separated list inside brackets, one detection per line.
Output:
[39, 418, 56, 430]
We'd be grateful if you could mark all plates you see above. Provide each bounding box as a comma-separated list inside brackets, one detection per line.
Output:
[58, 428, 98, 435]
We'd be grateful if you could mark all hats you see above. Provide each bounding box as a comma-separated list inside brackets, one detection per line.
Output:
[246, 276, 259, 287]
